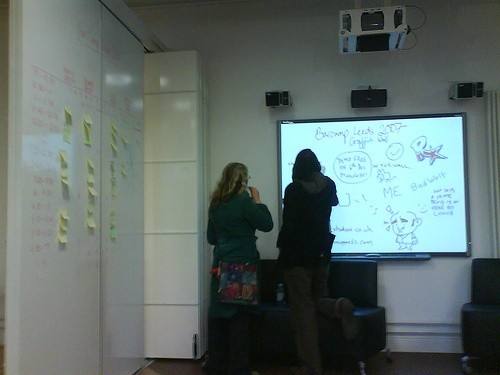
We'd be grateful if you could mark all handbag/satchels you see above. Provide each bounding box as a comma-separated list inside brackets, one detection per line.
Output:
[215, 260, 260, 306]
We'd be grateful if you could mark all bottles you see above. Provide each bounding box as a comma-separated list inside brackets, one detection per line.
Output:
[276, 283, 285, 306]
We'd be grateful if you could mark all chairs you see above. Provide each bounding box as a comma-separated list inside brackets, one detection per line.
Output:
[461, 258, 500, 375]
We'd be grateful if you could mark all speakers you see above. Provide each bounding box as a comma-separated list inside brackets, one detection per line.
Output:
[351, 89, 387, 108]
[449, 82, 484, 100]
[266, 91, 290, 107]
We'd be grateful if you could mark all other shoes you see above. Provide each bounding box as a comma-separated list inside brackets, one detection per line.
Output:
[288, 366, 300, 375]
[336, 298, 357, 341]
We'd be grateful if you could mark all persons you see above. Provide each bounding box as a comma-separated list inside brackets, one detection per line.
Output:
[207, 163, 273, 375]
[276, 148, 357, 375]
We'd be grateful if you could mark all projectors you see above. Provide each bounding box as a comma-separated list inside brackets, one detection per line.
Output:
[339, 4, 407, 55]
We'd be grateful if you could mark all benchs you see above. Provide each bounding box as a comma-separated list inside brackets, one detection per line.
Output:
[204, 258, 392, 375]
